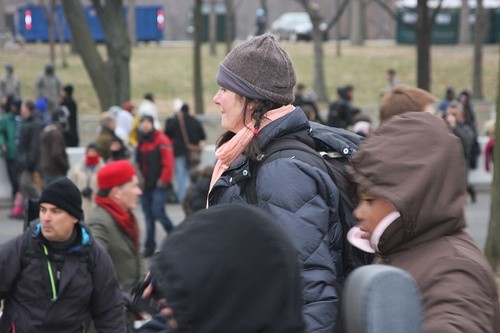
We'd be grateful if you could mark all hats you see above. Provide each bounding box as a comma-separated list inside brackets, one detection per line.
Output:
[216, 34, 297, 104]
[338, 84, 352, 92]
[352, 121, 370, 135]
[63, 85, 73, 93]
[38, 176, 84, 222]
[96, 160, 136, 189]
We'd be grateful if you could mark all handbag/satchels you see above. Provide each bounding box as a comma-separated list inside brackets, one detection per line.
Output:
[185, 143, 202, 168]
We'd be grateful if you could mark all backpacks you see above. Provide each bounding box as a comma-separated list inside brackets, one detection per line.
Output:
[245, 138, 369, 276]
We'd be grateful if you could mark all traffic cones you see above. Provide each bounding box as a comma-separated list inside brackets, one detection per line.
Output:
[9, 191, 25, 219]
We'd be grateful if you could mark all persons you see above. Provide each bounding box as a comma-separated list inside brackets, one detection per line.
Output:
[1, 178, 128, 333]
[205, 34, 364, 333]
[89, 160, 145, 299]
[379, 86, 442, 126]
[343, 112, 500, 332]
[130, 203, 306, 333]
[0, 64, 500, 251]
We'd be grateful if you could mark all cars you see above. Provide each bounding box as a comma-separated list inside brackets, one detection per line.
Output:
[268, 11, 331, 43]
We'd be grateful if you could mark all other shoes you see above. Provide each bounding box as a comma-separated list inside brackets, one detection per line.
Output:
[468, 189, 477, 203]
[8, 212, 24, 220]
[143, 244, 156, 257]
